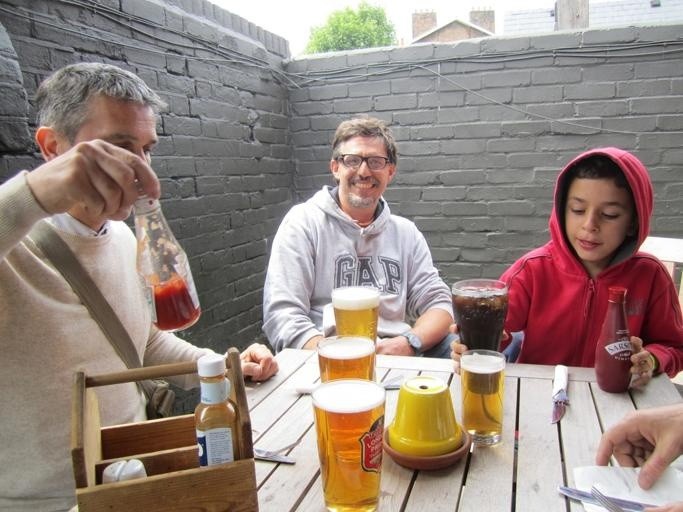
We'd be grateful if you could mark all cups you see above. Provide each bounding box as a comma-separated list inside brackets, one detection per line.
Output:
[459, 350, 508, 449]
[310, 334, 387, 512]
[449, 277, 510, 396]
[330, 284, 381, 346]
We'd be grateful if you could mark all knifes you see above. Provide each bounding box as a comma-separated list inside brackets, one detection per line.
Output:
[550, 365, 566, 424]
[557, 486, 660, 511]
[253, 448, 296, 464]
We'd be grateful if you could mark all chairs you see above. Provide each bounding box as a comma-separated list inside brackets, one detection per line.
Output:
[637, 235, 683, 313]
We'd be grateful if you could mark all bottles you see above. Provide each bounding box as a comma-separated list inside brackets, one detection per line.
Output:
[593, 283, 635, 395]
[131, 175, 202, 335]
[193, 354, 243, 469]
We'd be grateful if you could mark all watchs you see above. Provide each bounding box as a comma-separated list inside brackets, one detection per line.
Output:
[401, 330, 424, 357]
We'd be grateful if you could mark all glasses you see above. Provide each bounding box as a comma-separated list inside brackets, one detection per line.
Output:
[335, 154, 391, 170]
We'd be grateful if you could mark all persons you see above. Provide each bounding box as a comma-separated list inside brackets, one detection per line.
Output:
[444, 143, 682, 392]
[592, 402, 683, 512]
[0, 58, 282, 512]
[259, 115, 460, 361]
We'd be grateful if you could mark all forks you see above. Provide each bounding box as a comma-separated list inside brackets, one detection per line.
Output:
[590, 485, 626, 512]
